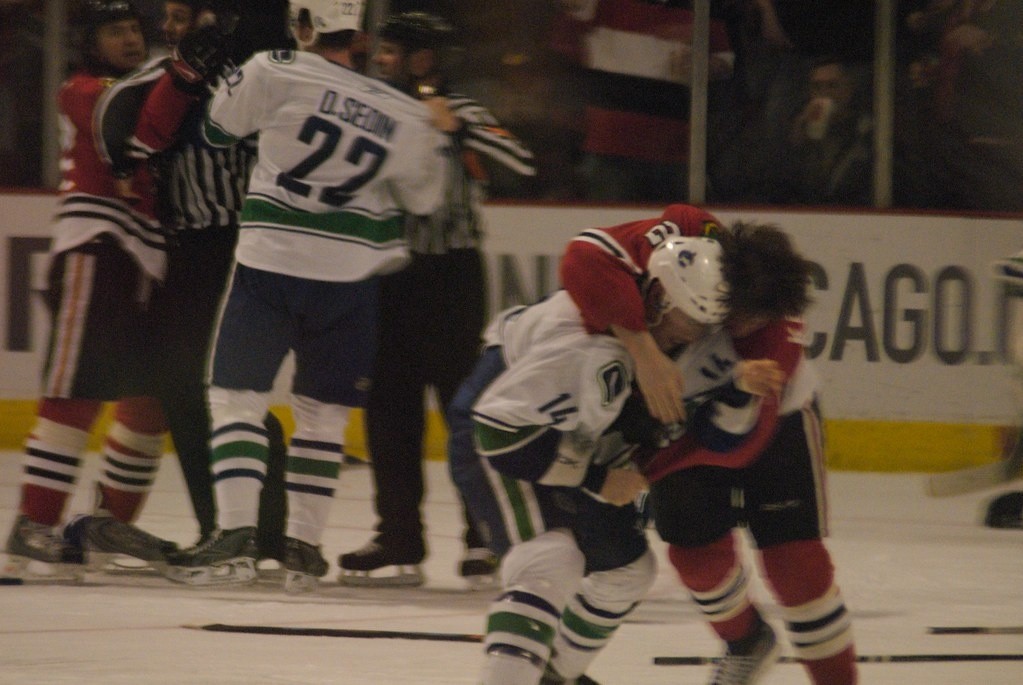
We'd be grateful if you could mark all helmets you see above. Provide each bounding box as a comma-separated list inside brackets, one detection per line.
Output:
[286, 0, 367, 50]
[645, 234, 732, 340]
[376, 13, 455, 66]
[69, 0, 141, 56]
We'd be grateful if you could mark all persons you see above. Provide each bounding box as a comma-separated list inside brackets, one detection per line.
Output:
[0, 0, 535, 591]
[448, 207, 859, 685]
[546, 0, 993, 211]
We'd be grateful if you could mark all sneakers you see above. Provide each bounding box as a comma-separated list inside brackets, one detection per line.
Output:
[0, 517, 81, 585]
[81, 515, 179, 583]
[163, 525, 258, 588]
[337, 528, 426, 586]
[711, 620, 782, 685]
[284, 536, 331, 597]
[460, 534, 512, 592]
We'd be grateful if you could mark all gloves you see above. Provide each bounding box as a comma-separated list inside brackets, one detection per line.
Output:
[178, 3, 245, 83]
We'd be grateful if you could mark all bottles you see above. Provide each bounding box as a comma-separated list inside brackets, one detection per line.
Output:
[808, 97, 833, 138]
